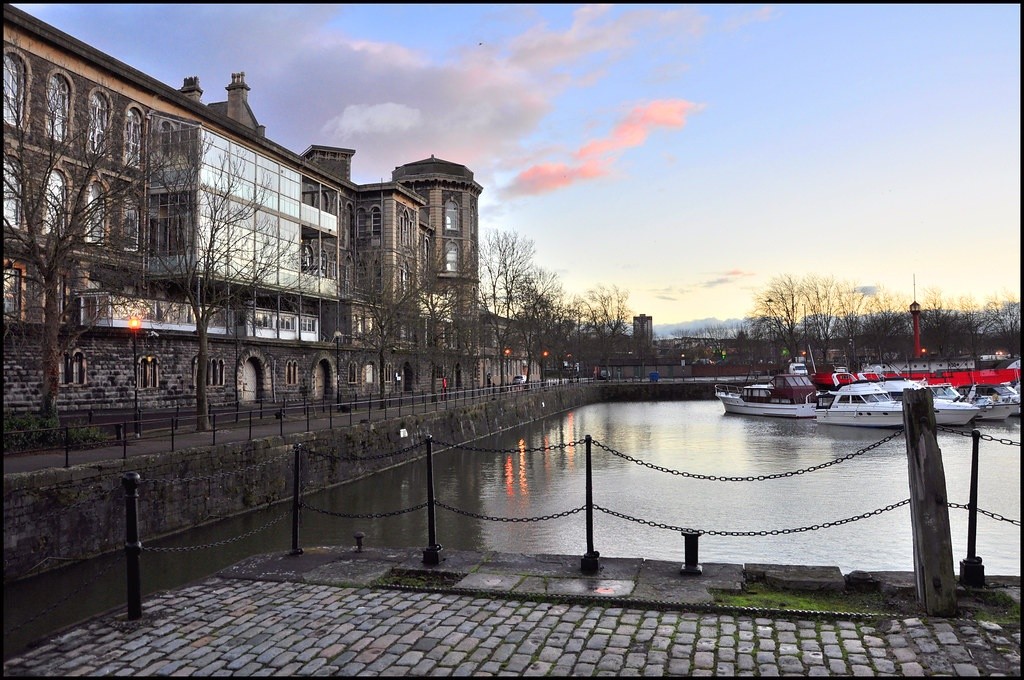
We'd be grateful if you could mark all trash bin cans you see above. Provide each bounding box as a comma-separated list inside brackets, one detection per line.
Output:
[649, 372, 659, 382]
[340, 394, 351, 413]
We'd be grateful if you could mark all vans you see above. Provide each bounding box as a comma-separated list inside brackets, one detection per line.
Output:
[789, 362, 808, 377]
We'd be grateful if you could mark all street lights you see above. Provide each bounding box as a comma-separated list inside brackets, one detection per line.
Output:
[682, 354, 685, 382]
[567, 354, 572, 382]
[544, 351, 548, 387]
[334, 330, 342, 412]
[131, 319, 140, 439]
[505, 349, 510, 391]
[802, 351, 806, 376]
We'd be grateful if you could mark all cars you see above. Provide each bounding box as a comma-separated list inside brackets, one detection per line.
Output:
[599, 370, 611, 379]
[513, 375, 531, 384]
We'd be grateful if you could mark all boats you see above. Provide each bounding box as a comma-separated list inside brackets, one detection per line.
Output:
[714, 374, 818, 419]
[816, 378, 1021, 427]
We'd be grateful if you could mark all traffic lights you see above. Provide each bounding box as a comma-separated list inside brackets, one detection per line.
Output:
[721, 355, 726, 361]
